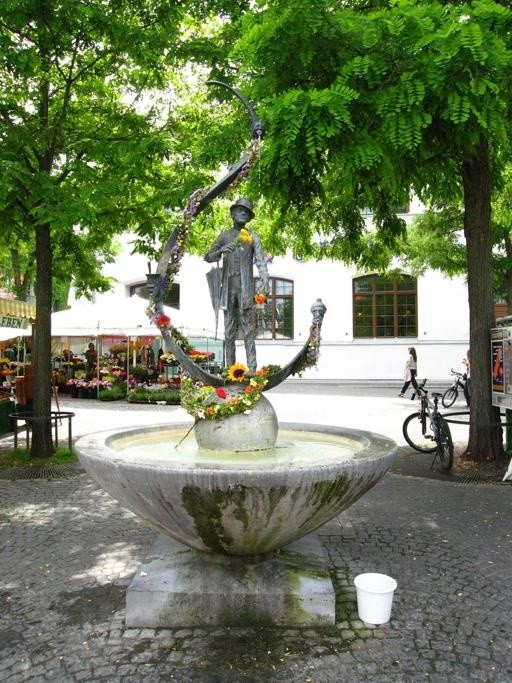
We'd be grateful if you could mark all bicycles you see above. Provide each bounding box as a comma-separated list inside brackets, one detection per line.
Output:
[441, 367, 471, 408]
[402, 377, 455, 472]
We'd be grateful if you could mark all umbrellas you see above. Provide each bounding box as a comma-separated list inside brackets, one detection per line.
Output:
[204, 258, 227, 342]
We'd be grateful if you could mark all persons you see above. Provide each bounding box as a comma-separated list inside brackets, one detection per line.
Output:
[398, 346, 424, 400]
[309, 297, 328, 327]
[204, 195, 272, 376]
[85, 342, 99, 373]
[461, 346, 471, 408]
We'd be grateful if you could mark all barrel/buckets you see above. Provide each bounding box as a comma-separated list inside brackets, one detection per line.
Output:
[355, 573, 397, 624]
